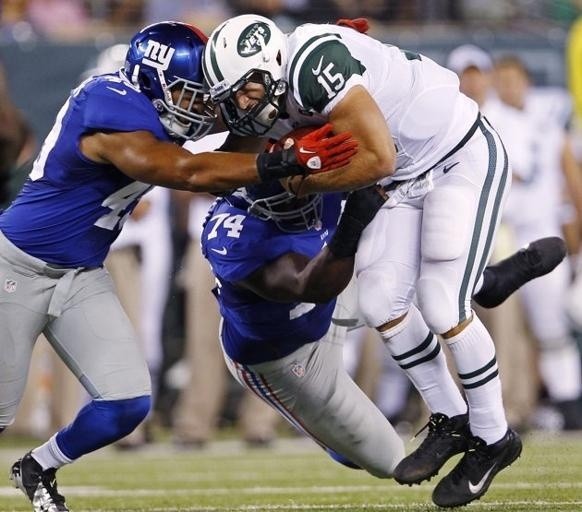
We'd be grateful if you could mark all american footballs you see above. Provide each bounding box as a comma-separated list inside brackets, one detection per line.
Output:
[273, 125, 334, 151]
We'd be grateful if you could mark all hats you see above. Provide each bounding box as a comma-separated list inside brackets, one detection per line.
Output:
[445, 43, 493, 73]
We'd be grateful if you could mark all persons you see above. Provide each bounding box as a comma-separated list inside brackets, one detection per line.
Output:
[2, 2, 581, 452]
[202, 11, 523, 509]
[200, 184, 570, 481]
[0, 17, 372, 512]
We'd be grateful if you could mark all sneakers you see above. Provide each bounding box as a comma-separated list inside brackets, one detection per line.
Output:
[474, 237, 566, 308]
[431, 428, 521, 508]
[393, 409, 470, 485]
[9, 454, 69, 507]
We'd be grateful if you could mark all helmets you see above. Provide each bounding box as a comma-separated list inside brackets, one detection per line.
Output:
[220, 133, 324, 235]
[199, 14, 286, 138]
[120, 20, 217, 143]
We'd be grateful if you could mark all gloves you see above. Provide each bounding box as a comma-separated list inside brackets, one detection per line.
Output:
[257, 125, 360, 177]
[336, 14, 370, 36]
